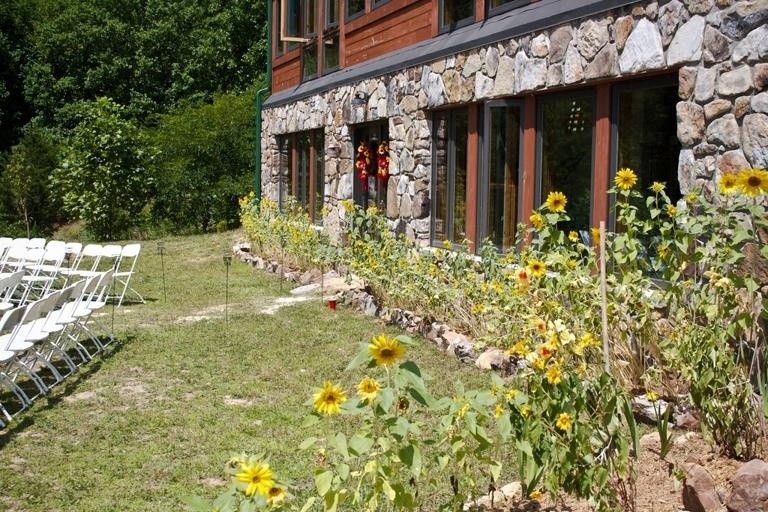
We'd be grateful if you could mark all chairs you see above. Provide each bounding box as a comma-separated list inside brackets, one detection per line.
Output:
[0, 235, 146, 443]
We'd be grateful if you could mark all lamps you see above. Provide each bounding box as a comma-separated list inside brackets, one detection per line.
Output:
[350, 88, 379, 113]
[327, 128, 343, 157]
